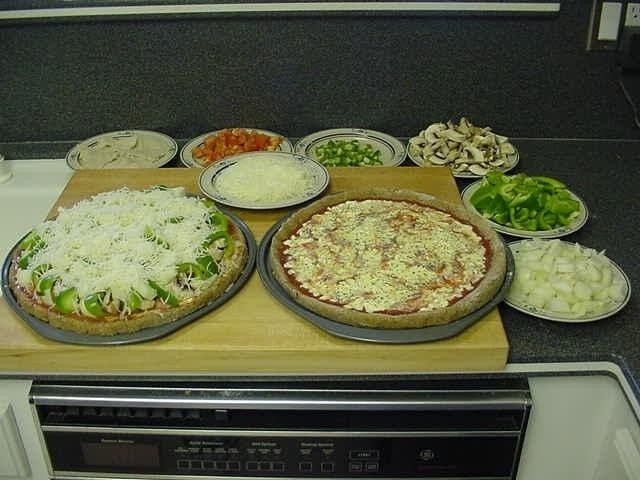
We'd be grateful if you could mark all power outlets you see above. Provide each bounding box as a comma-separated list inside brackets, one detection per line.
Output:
[624, 3, 640, 27]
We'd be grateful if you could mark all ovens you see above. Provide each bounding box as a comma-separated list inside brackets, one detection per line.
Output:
[32, 380, 535, 479]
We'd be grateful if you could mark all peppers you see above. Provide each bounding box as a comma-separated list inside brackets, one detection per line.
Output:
[469, 172, 581, 231]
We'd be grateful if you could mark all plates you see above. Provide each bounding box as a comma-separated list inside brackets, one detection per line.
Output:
[293, 127, 406, 166]
[66, 130, 180, 169]
[181, 127, 293, 168]
[197, 151, 330, 211]
[501, 236, 631, 324]
[406, 126, 520, 173]
[460, 172, 589, 237]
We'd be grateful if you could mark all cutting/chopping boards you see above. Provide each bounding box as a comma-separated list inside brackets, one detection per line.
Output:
[1, 166, 511, 374]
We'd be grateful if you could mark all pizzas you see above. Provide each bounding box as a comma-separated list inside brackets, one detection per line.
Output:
[269, 189, 508, 328]
[8, 187, 251, 335]
